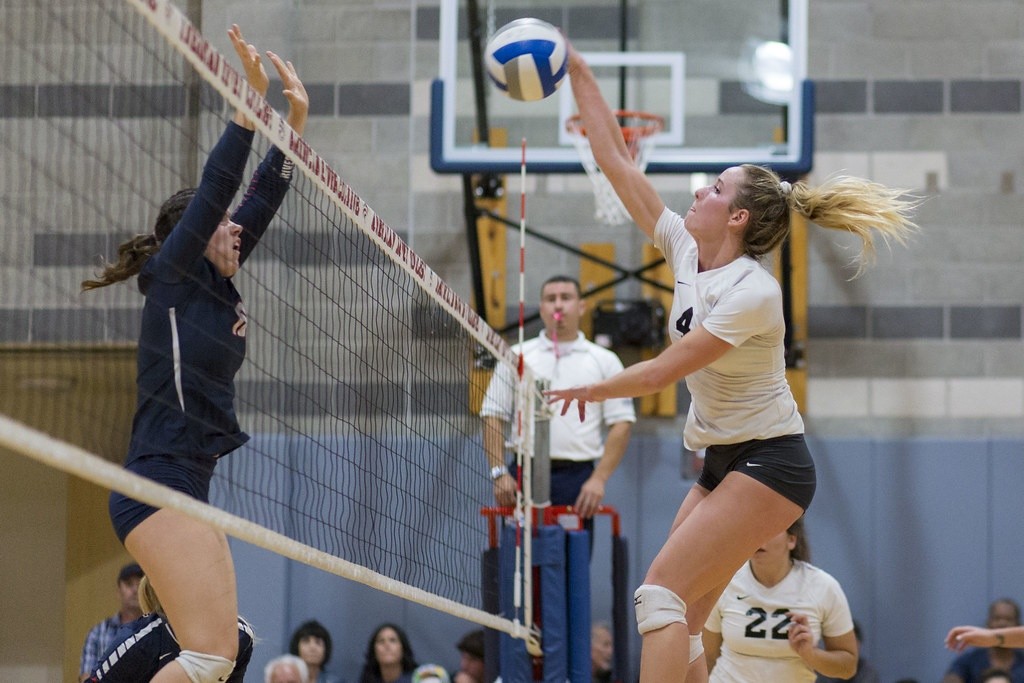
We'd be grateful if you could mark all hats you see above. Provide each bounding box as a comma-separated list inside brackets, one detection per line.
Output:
[118, 563, 142, 579]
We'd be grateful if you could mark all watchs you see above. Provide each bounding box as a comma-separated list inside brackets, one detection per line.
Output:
[489, 465, 508, 480]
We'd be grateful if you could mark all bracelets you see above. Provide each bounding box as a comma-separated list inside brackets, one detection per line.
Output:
[993, 628, 1004, 648]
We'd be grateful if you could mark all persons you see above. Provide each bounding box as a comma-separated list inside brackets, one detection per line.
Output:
[541, 42, 921, 683]
[75, 519, 1024, 683]
[478, 275, 636, 592]
[78, 23, 311, 683]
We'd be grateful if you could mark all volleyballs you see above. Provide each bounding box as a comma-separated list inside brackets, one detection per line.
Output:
[482, 14, 570, 103]
